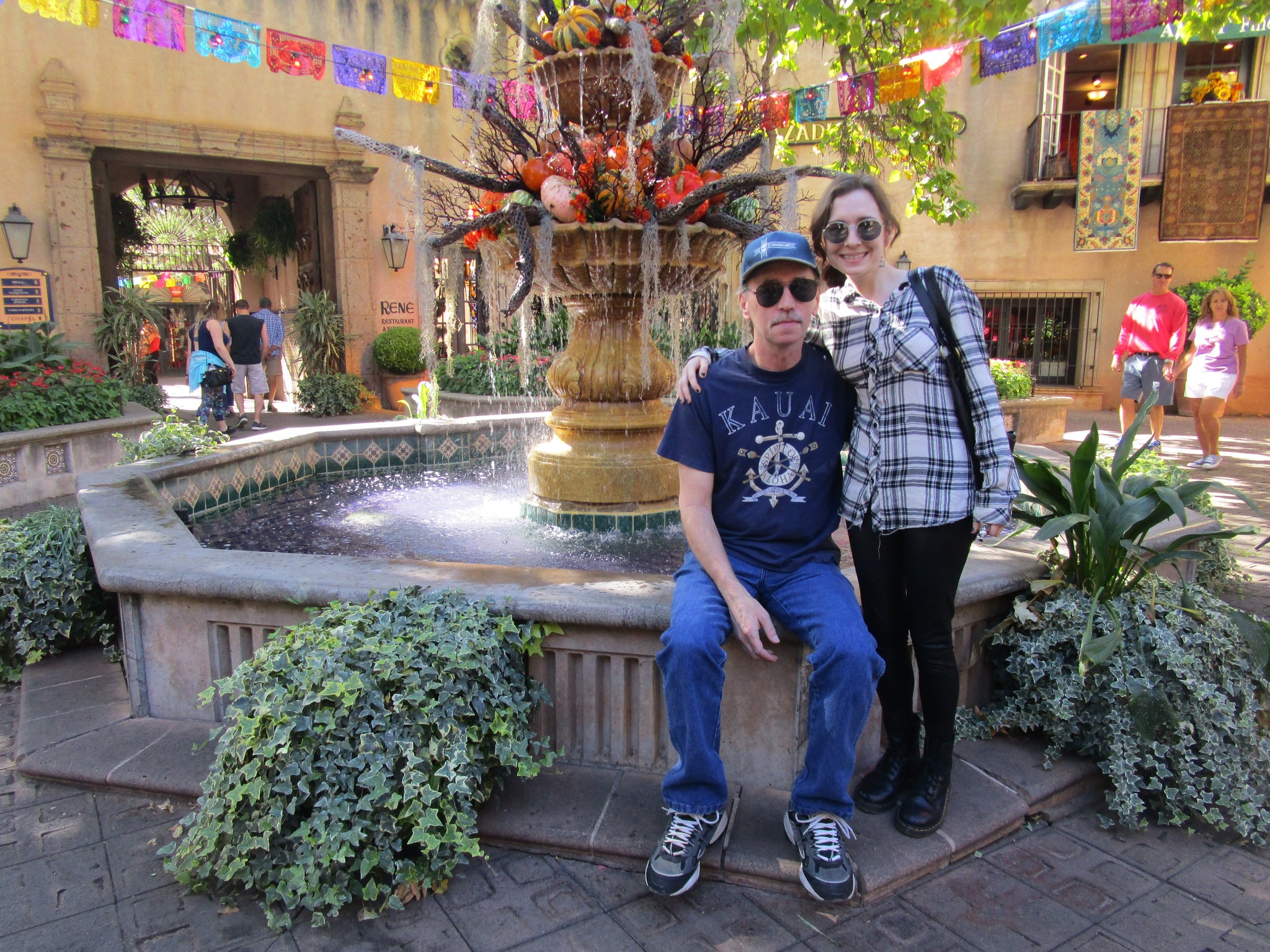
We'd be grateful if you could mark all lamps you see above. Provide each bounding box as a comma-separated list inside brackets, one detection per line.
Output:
[1, 204, 34, 264]
[139, 169, 235, 219]
[1087, 75, 1109, 100]
[379, 223, 411, 273]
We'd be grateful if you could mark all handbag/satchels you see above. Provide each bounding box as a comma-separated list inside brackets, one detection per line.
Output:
[906, 268, 1017, 492]
[205, 367, 233, 388]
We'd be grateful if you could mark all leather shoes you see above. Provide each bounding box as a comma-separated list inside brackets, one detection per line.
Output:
[853, 748, 920, 815]
[895, 765, 951, 838]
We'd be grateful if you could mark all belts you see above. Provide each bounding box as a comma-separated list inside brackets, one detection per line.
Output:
[1134, 351, 1159, 357]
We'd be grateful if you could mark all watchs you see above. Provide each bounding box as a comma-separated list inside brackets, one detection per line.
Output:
[1164, 358, 1175, 364]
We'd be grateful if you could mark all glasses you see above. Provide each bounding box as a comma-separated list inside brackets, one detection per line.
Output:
[1154, 273, 1172, 279]
[747, 277, 818, 308]
[823, 218, 886, 244]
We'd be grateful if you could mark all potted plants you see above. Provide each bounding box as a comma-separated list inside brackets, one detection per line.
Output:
[377, 326, 434, 412]
[256, 194, 298, 263]
[1165, 256, 1269, 416]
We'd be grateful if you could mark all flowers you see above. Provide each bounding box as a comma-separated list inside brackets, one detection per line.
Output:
[1191, 71, 1230, 105]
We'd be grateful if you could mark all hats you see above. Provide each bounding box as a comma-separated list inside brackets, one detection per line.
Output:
[740, 231, 821, 284]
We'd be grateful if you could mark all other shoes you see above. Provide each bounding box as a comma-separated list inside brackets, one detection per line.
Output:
[252, 422, 267, 430]
[228, 406, 235, 415]
[225, 411, 230, 417]
[203, 434, 212, 439]
[267, 405, 278, 412]
[220, 426, 237, 437]
[236, 414, 248, 429]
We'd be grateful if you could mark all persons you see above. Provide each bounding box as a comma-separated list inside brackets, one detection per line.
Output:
[645, 233, 890, 903]
[1163, 287, 1249, 470]
[676, 175, 1022, 840]
[1111, 262, 1189, 457]
[134, 297, 285, 436]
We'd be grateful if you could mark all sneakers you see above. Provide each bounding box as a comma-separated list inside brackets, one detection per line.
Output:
[1104, 436, 1134, 452]
[1201, 455, 1222, 470]
[1145, 440, 1162, 453]
[645, 803, 729, 896]
[783, 807, 858, 903]
[1187, 456, 1203, 468]
